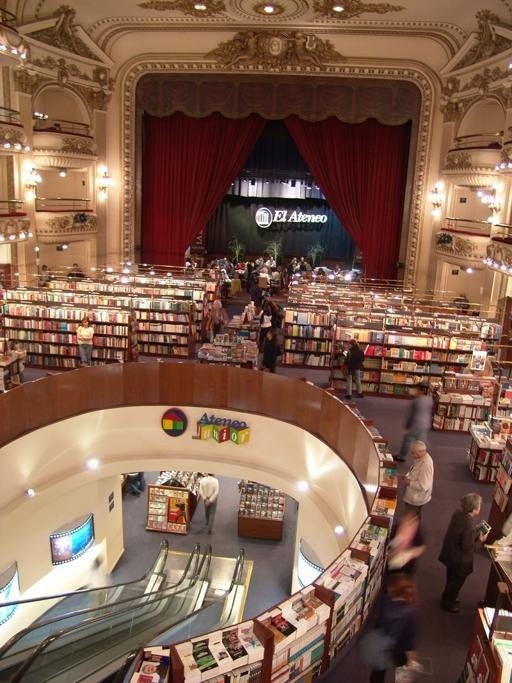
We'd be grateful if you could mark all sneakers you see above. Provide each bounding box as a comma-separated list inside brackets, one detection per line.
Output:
[455, 598, 462, 602]
[451, 608, 459, 612]
[345, 395, 352, 399]
[393, 455, 405, 462]
[359, 393, 364, 397]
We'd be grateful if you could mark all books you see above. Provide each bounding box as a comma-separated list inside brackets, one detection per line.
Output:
[3, 275, 511, 683]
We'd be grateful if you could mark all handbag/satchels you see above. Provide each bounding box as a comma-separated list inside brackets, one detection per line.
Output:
[395, 662, 423, 683]
[276, 344, 284, 355]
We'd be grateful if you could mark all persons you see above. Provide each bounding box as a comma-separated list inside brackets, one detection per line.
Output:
[210, 255, 326, 293]
[40, 265, 51, 286]
[67, 263, 84, 282]
